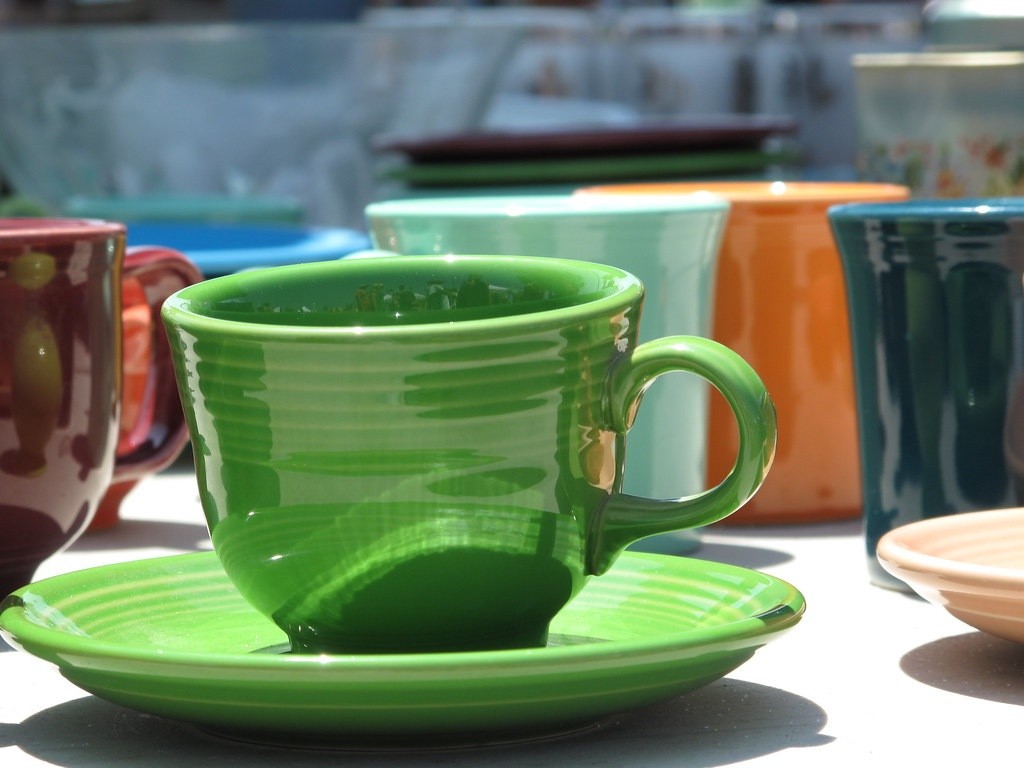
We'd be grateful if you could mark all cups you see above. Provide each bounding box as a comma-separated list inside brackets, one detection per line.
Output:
[364, 191, 732, 502]
[0, 218, 205, 568]
[826, 197, 1024, 593]
[582, 182, 909, 524]
[159, 253, 779, 657]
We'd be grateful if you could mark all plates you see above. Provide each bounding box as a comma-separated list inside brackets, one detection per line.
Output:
[874, 506, 1024, 643]
[368, 112, 803, 188]
[0, 551, 808, 742]
